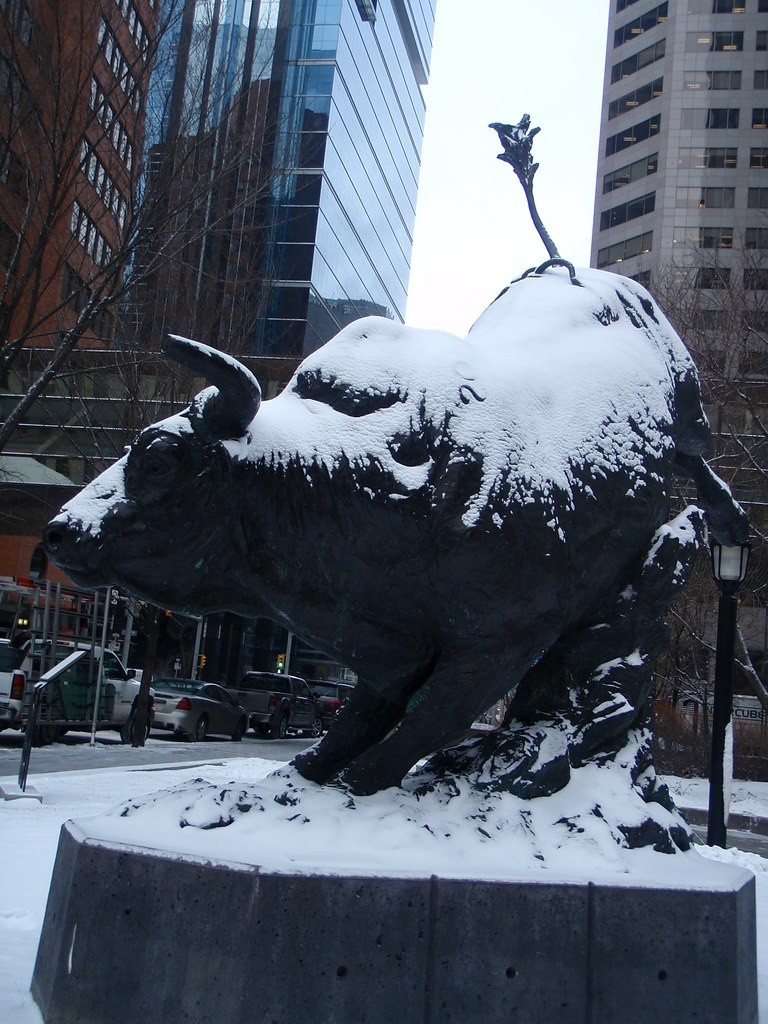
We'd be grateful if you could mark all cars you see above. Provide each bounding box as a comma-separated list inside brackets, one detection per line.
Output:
[146, 677, 249, 743]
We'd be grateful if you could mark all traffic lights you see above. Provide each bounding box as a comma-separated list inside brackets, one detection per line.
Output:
[198, 654, 208, 668]
[276, 654, 287, 670]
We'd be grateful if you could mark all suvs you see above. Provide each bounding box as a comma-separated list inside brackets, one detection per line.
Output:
[123, 668, 155, 687]
[305, 678, 358, 729]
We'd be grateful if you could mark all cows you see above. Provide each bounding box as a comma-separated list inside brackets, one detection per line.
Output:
[38, 112, 753, 846]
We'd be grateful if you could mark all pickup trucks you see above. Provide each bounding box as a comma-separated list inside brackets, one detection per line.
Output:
[0, 630, 156, 752]
[224, 671, 325, 740]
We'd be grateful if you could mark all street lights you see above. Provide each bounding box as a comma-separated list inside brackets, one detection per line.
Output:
[703, 523, 752, 851]
